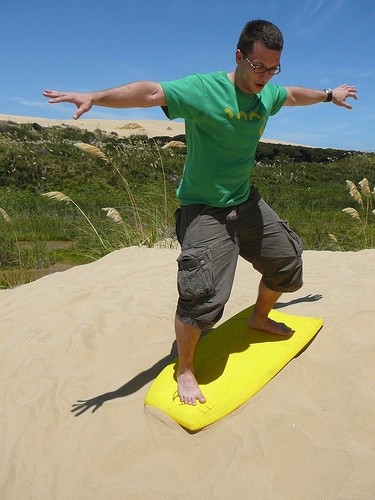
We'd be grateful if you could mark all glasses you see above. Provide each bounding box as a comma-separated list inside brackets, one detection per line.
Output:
[238, 48, 281, 76]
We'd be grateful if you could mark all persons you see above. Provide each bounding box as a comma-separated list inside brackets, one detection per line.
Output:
[43, 19, 357, 405]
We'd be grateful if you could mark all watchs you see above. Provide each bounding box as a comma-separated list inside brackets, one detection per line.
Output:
[323, 87, 333, 102]
